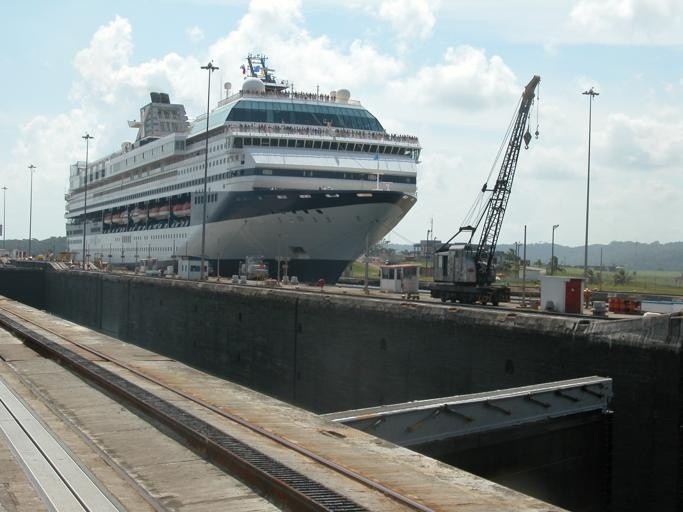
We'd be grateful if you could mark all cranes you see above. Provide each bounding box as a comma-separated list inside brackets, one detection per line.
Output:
[427, 75, 541, 306]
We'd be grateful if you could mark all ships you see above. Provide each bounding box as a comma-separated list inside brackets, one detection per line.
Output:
[64, 53, 421, 285]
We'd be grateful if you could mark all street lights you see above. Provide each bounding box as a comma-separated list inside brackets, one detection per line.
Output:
[550, 224, 560, 275]
[26, 163, 35, 256]
[0, 186, 7, 248]
[199, 62, 218, 279]
[81, 132, 94, 270]
[581, 86, 599, 289]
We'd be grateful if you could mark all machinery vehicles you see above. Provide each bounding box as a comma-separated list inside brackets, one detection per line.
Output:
[239, 255, 268, 279]
